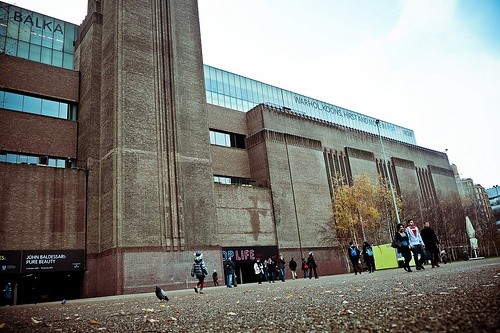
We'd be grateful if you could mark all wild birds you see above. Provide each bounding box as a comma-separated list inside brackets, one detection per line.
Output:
[61, 299, 66, 305]
[155, 285, 169, 302]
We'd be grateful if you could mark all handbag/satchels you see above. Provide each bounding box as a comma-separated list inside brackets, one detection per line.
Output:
[391, 238, 402, 248]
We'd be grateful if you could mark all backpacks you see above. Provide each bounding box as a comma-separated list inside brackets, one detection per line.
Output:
[365, 246, 373, 256]
[349, 246, 357, 256]
[303, 261, 309, 271]
[226, 260, 232, 270]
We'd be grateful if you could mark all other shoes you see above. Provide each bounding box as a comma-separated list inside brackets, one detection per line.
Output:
[433, 262, 440, 267]
[227, 285, 229, 287]
[200, 289, 203, 293]
[402, 264, 407, 271]
[229, 286, 232, 288]
[407, 266, 413, 272]
[194, 286, 198, 293]
[431, 265, 435, 268]
[416, 266, 421, 270]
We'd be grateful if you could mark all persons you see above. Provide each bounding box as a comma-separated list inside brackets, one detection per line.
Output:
[289, 257, 298, 280]
[405, 221, 427, 271]
[391, 224, 414, 273]
[420, 221, 442, 268]
[301, 258, 309, 278]
[348, 241, 362, 275]
[361, 241, 375, 273]
[307, 252, 319, 279]
[224, 256, 235, 289]
[277, 254, 286, 282]
[254, 258, 265, 284]
[212, 270, 220, 286]
[266, 257, 276, 283]
[191, 252, 208, 294]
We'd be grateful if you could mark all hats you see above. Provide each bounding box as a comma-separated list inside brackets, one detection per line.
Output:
[194, 252, 203, 257]
